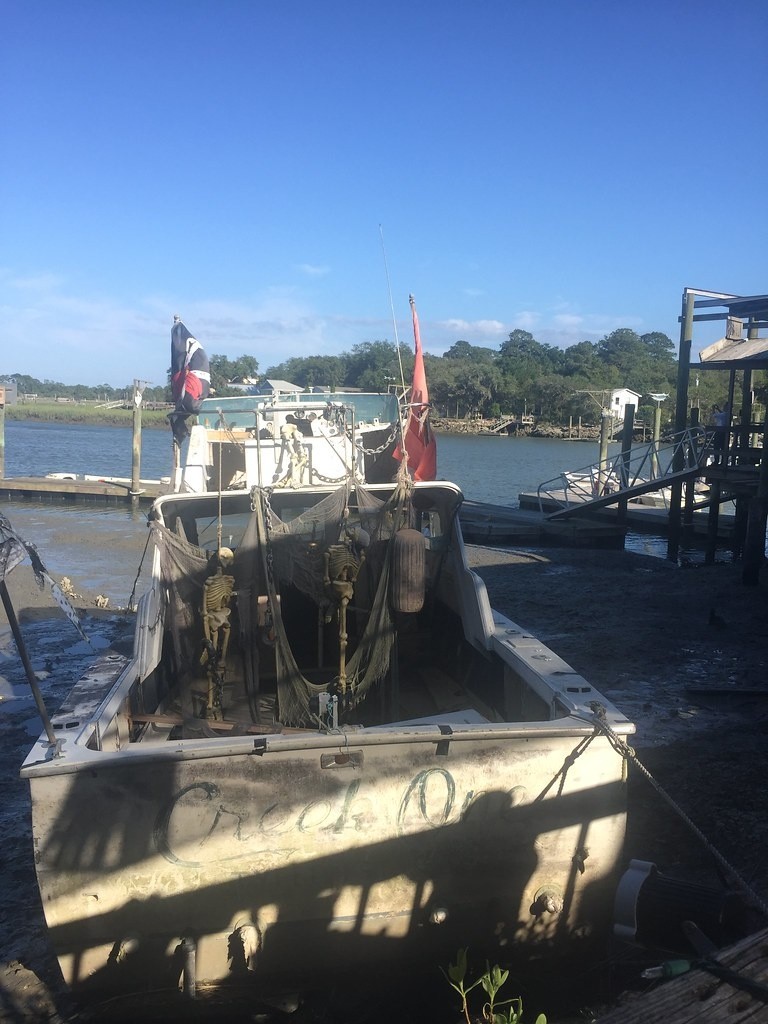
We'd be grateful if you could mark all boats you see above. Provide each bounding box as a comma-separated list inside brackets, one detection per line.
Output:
[0, 291, 768, 1024]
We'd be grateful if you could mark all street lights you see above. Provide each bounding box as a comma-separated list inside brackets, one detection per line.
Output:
[645, 392, 669, 494]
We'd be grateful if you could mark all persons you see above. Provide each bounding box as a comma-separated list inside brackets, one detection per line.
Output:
[280, 415, 297, 438]
[309, 413, 323, 436]
[712, 402, 731, 465]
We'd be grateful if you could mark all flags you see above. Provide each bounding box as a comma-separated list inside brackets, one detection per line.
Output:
[166, 320, 210, 445]
[393, 303, 437, 480]
